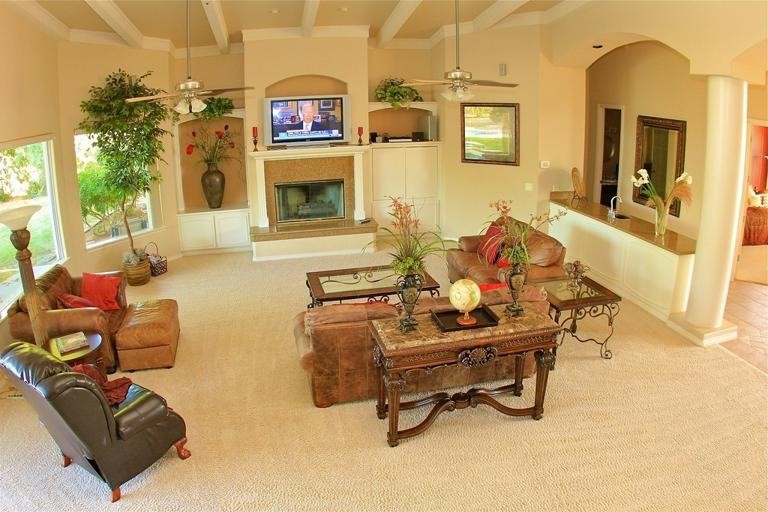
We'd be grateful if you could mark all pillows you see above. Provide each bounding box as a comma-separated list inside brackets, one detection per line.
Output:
[18, 264, 122, 312]
[476, 223, 519, 269]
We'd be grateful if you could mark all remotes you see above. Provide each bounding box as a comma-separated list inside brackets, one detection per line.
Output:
[359, 218, 371, 224]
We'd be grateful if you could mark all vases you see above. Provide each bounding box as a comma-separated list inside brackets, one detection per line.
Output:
[655, 206, 670, 237]
[201, 163, 225, 208]
[395, 274, 423, 331]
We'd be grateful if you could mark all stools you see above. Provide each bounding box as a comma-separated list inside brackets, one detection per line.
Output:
[114, 299, 179, 373]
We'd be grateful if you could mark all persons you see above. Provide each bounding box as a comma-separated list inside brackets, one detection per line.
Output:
[292, 103, 330, 130]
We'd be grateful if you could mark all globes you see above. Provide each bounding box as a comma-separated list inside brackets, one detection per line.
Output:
[448, 279, 481, 326]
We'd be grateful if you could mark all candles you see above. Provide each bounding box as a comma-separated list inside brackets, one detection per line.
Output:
[358, 127, 363, 136]
[253, 127, 257, 137]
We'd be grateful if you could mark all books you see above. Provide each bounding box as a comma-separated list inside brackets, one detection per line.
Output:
[55, 330, 89, 353]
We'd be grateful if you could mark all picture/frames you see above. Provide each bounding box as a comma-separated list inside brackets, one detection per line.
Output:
[460, 103, 520, 166]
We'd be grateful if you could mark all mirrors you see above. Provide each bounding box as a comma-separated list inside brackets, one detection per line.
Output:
[632, 115, 687, 218]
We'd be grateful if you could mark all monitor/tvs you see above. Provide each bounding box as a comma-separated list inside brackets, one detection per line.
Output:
[264, 94, 351, 149]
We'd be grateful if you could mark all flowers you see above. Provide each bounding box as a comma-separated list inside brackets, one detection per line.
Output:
[186, 124, 242, 171]
[631, 169, 693, 235]
[387, 195, 425, 275]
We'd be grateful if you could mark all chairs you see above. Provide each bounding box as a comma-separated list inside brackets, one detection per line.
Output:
[0, 340, 192, 503]
[7, 271, 127, 374]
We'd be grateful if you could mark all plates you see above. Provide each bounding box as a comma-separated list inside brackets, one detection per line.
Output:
[571, 167, 585, 199]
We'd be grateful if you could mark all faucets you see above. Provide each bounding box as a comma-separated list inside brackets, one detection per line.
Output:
[608, 196, 622, 217]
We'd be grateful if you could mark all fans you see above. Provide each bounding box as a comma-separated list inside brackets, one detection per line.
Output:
[125, 0, 255, 103]
[398, 0, 519, 87]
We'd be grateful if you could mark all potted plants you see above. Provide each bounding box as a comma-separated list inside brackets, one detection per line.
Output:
[79, 69, 180, 285]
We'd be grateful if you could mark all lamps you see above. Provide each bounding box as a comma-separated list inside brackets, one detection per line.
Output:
[173, 94, 207, 114]
[0, 205, 49, 347]
[440, 83, 475, 102]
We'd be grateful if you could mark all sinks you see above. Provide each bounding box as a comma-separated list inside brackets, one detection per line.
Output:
[614, 214, 632, 221]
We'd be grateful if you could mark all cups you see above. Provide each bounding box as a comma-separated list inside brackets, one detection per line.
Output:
[412, 132, 424, 141]
[369, 131, 390, 143]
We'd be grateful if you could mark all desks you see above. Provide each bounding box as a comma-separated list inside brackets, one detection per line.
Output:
[367, 301, 563, 447]
[42, 331, 103, 367]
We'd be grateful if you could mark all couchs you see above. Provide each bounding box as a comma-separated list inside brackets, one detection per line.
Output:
[445, 216, 565, 291]
[299, 284, 562, 408]
